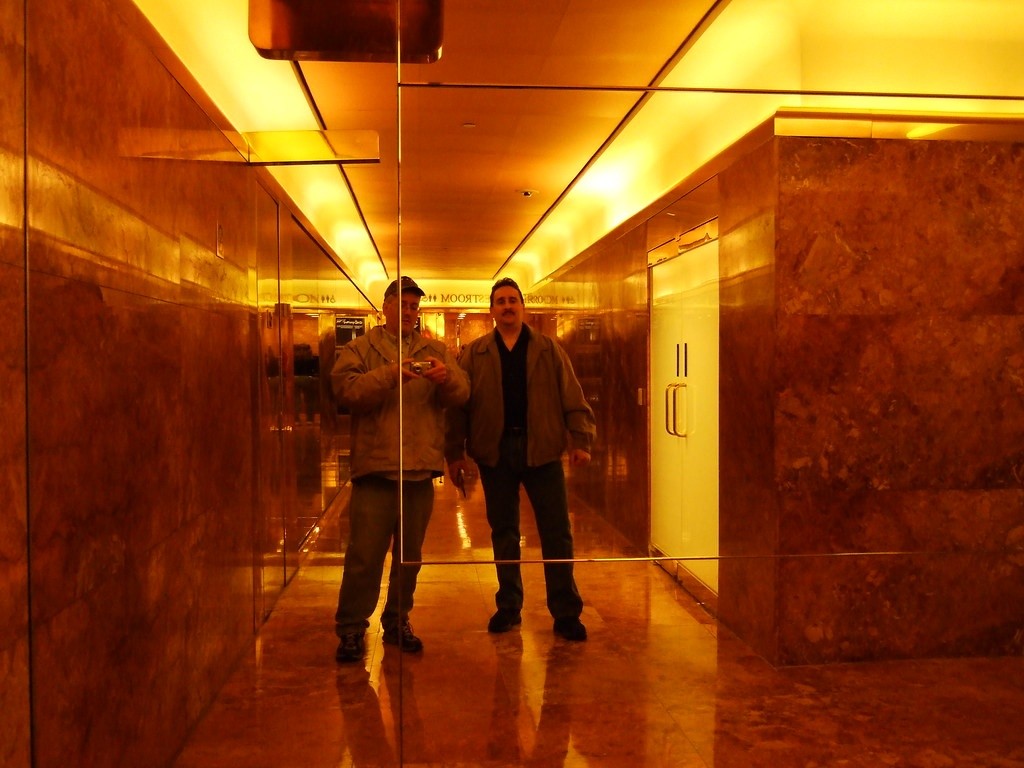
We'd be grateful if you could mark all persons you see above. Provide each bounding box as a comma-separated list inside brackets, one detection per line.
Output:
[330, 276, 470, 664]
[446, 278, 596, 640]
[294, 343, 318, 424]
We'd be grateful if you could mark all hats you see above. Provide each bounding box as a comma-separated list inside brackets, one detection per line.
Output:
[383, 276, 425, 297]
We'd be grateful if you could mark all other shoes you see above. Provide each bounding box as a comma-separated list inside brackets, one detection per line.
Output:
[552, 617, 588, 641]
[485, 605, 522, 634]
[383, 623, 422, 652]
[334, 627, 366, 662]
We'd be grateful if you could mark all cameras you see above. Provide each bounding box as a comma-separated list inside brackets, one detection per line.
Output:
[410, 361, 432, 375]
